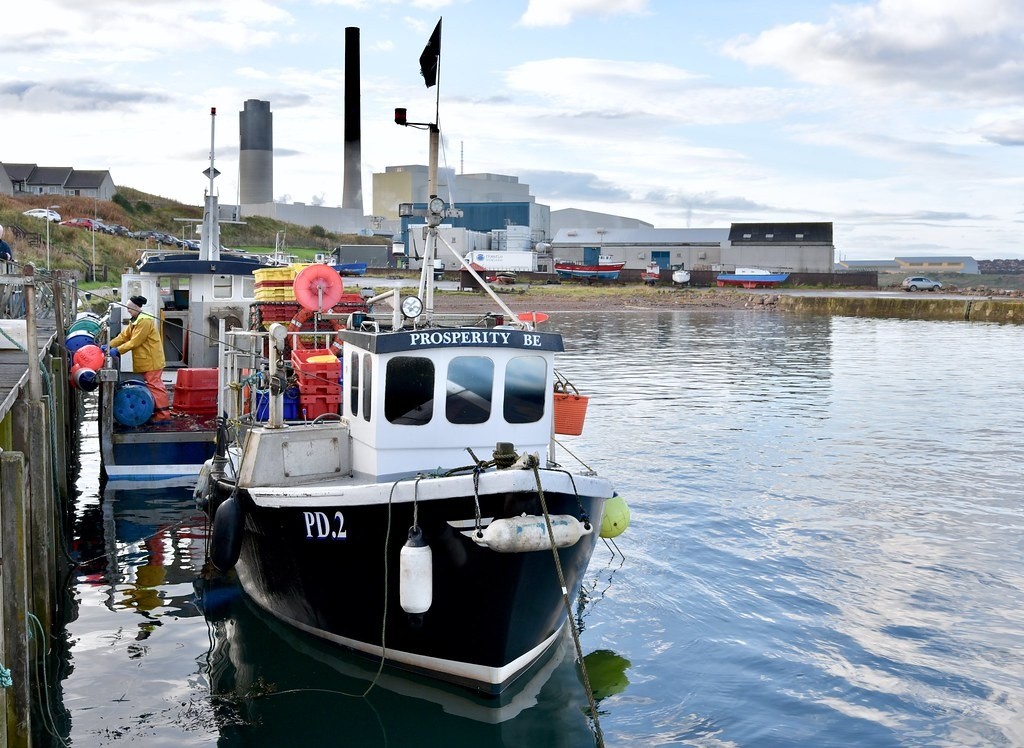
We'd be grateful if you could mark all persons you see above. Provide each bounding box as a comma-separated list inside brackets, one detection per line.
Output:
[101, 296, 173, 424]
[0, 224, 12, 274]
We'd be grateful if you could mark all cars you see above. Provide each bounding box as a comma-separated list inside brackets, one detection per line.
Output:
[59, 217, 230, 253]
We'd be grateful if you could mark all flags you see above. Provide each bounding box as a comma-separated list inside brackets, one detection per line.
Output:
[419, 16, 442, 88]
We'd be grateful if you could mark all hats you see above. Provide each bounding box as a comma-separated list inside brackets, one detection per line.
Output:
[130, 295, 148, 307]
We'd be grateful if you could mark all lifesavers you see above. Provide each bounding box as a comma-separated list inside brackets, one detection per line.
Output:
[287, 307, 348, 356]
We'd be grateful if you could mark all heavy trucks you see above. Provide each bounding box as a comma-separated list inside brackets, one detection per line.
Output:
[327, 245, 388, 269]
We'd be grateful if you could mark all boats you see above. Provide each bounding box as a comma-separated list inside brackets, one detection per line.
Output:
[554, 262, 625, 280]
[717, 267, 792, 287]
[641, 261, 661, 283]
[97, 106, 370, 490]
[671, 271, 690, 283]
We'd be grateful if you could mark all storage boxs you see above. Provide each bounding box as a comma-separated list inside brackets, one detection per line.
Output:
[172, 369, 218, 414]
[243, 268, 369, 421]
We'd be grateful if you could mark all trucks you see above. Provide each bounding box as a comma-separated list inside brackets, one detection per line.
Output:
[460, 250, 538, 273]
[190, 107, 615, 725]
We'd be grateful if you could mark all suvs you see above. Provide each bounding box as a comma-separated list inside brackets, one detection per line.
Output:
[901, 276, 943, 292]
[22, 209, 61, 223]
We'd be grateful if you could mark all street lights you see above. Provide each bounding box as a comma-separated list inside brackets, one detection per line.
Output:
[274, 229, 285, 266]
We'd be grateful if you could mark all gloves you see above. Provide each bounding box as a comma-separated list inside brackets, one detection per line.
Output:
[151, 417, 173, 425]
[101, 345, 108, 351]
[110, 347, 119, 358]
